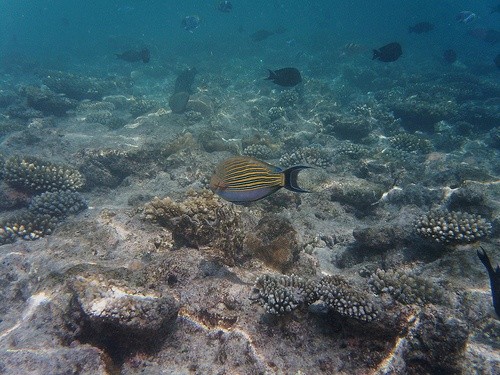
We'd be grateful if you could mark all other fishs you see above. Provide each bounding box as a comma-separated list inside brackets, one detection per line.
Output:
[442, 48, 460, 66]
[371, 42, 405, 64]
[261, 67, 303, 88]
[250, 29, 275, 42]
[408, 20, 437, 35]
[207, 153, 318, 207]
[179, 14, 209, 35]
[214, 0, 234, 14]
[456, 3, 500, 45]
[493, 53, 500, 69]
[113, 43, 162, 66]
